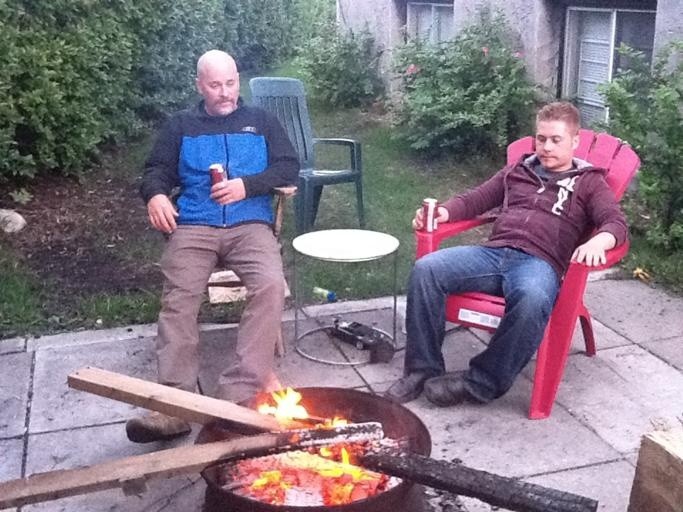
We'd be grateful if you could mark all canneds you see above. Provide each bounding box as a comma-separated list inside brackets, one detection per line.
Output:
[421, 198, 438, 232]
[209, 164, 227, 199]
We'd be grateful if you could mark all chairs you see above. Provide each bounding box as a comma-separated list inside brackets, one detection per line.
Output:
[158, 185, 300, 360]
[248, 77, 366, 235]
[412, 128, 641, 421]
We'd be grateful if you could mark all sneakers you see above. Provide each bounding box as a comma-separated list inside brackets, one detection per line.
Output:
[126, 410, 191, 443]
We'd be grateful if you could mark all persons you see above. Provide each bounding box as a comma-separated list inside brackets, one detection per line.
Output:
[383, 100, 628, 406]
[126, 49, 300, 443]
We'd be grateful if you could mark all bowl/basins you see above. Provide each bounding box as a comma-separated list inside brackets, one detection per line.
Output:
[192, 384, 434, 511]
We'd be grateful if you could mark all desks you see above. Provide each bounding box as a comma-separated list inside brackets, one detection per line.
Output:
[291, 229, 400, 365]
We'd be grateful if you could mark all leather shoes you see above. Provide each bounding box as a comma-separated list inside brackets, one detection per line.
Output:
[425, 372, 472, 406]
[385, 370, 425, 403]
